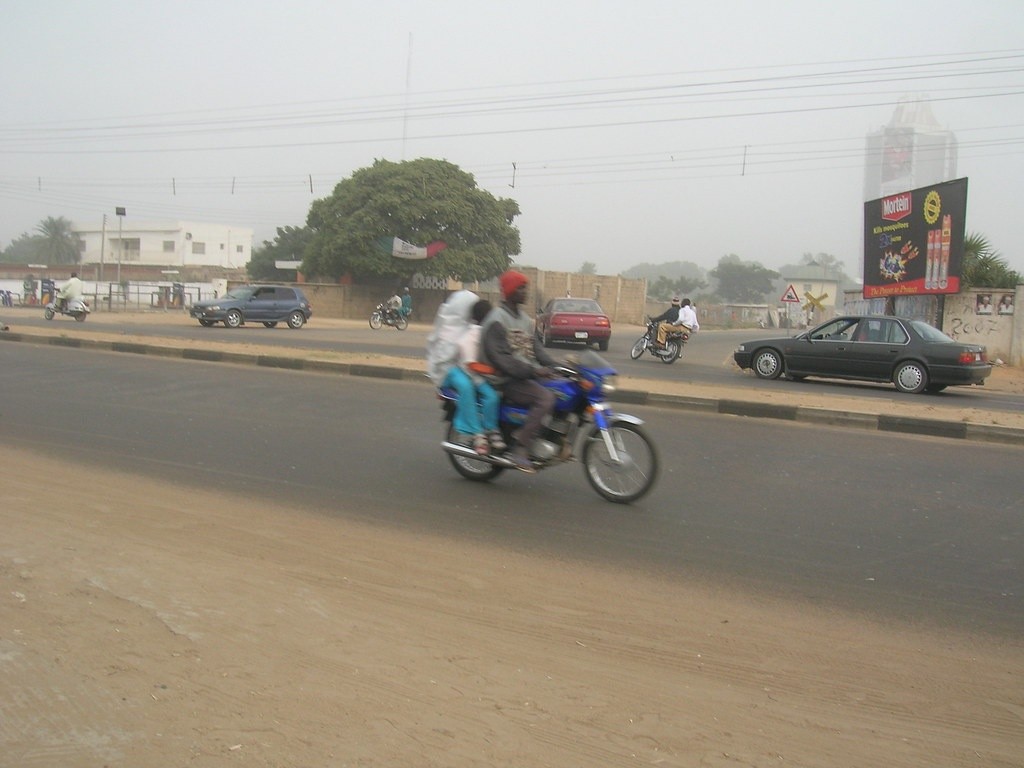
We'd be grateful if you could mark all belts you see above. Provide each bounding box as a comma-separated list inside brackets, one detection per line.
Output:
[680, 324, 691, 333]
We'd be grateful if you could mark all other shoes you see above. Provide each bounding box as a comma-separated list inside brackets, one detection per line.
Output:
[655, 341, 666, 349]
[473, 434, 492, 455]
[487, 428, 507, 450]
[502, 445, 536, 473]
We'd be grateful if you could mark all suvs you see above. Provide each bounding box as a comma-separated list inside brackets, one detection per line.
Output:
[190, 281, 313, 329]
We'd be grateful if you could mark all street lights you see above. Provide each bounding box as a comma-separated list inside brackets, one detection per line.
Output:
[115, 206, 127, 312]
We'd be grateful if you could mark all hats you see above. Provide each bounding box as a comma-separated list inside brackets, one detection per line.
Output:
[672, 297, 680, 305]
[502, 270, 528, 299]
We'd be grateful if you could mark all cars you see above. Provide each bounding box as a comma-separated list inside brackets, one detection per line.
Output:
[733, 312, 994, 394]
[534, 296, 612, 351]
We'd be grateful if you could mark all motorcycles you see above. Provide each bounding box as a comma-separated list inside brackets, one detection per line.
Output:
[368, 302, 411, 331]
[631, 315, 690, 366]
[44, 287, 91, 322]
[435, 347, 663, 506]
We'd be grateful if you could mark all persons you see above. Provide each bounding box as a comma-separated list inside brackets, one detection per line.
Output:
[479, 269, 562, 473]
[649, 296, 699, 350]
[426, 290, 508, 456]
[730, 311, 737, 323]
[387, 287, 412, 323]
[54, 272, 82, 309]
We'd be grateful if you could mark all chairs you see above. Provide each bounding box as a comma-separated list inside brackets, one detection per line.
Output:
[581, 306, 589, 312]
[870, 329, 879, 342]
[556, 304, 566, 312]
